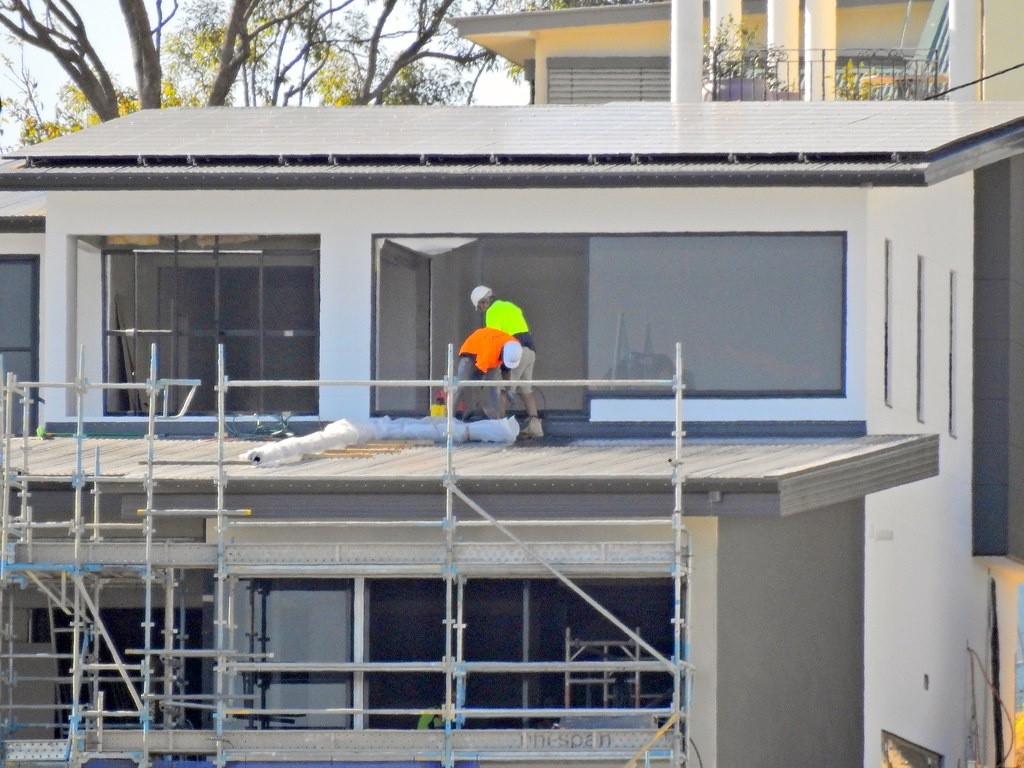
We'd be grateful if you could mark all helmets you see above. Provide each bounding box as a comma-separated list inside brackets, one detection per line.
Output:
[503, 341, 524, 370]
[470, 285, 493, 312]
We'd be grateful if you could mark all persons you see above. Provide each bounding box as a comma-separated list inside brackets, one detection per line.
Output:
[417, 688, 456, 729]
[451, 285, 545, 438]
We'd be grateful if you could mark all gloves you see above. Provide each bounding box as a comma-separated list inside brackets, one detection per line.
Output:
[506, 391, 516, 405]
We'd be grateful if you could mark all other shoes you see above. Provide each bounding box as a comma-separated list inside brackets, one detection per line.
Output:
[517, 416, 544, 440]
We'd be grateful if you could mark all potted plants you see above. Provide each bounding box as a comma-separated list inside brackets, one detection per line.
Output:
[704, 12, 802, 100]
